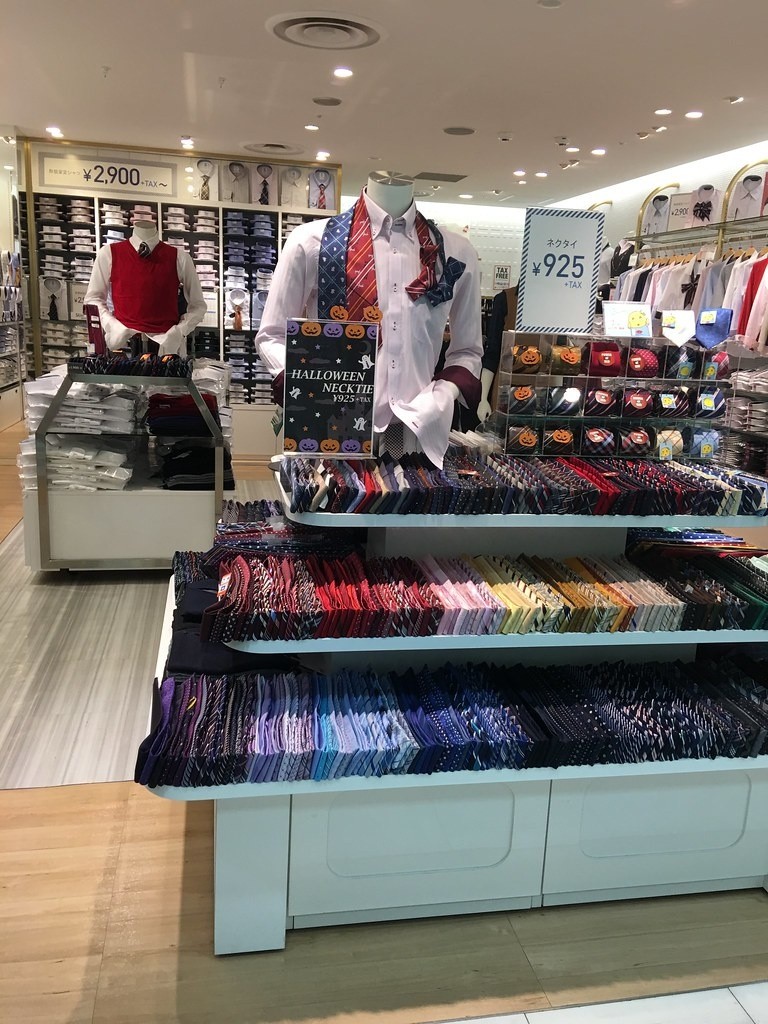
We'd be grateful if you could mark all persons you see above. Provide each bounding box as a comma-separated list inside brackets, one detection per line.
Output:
[255, 170, 484, 471]
[610, 239, 635, 278]
[84, 222, 208, 358]
[598, 237, 615, 285]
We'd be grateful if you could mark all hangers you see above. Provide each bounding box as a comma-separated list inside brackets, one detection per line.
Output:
[632, 232, 768, 271]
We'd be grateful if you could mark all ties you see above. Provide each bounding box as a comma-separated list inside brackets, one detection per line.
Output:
[346, 182, 440, 350]
[281, 452, 768, 516]
[384, 422, 424, 459]
[318, 184, 327, 209]
[233, 305, 243, 331]
[662, 310, 696, 348]
[431, 365, 482, 410]
[139, 242, 150, 257]
[695, 308, 733, 350]
[83, 354, 194, 379]
[199, 174, 211, 200]
[134, 659, 768, 789]
[317, 198, 467, 319]
[47, 294, 58, 321]
[259, 180, 269, 205]
[172, 499, 768, 646]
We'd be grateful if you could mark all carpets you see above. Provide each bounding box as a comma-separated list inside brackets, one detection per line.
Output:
[1, 479, 284, 791]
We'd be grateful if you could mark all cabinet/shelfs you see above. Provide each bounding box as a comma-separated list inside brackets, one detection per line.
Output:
[498, 328, 742, 463]
[150, 470, 768, 958]
[0, 137, 341, 573]
[719, 389, 768, 439]
